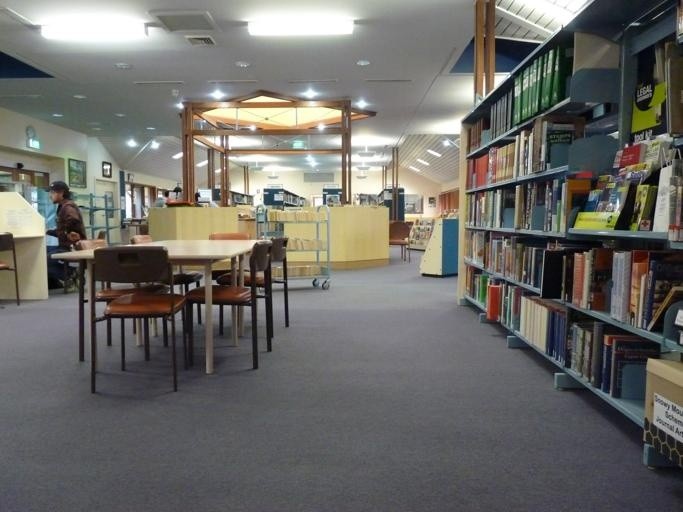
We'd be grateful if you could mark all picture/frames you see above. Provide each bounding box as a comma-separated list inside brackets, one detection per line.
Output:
[102, 161, 112, 178]
[69, 158, 86, 188]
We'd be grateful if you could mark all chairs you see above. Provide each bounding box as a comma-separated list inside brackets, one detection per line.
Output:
[63, 230, 107, 293]
[89, 245, 187, 393]
[186, 241, 272, 370]
[209, 233, 249, 281]
[75, 239, 168, 362]
[389, 222, 411, 263]
[129, 235, 203, 325]
[0, 232, 20, 305]
[217, 237, 290, 337]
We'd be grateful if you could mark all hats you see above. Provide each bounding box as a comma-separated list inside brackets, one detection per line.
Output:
[45, 181, 68, 192]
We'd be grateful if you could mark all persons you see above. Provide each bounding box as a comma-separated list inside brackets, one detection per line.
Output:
[45, 181, 86, 293]
[173, 187, 182, 199]
[163, 191, 169, 207]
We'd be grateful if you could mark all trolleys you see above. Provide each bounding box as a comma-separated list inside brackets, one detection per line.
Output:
[249, 201, 337, 295]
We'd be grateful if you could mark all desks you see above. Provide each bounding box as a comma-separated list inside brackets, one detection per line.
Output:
[51, 239, 272, 374]
[0, 190, 47, 301]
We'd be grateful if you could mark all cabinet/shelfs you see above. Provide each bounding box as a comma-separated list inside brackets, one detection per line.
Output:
[255, 204, 332, 289]
[198, 187, 404, 218]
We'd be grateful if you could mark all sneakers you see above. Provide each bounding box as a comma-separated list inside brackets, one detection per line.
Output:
[64, 267, 85, 293]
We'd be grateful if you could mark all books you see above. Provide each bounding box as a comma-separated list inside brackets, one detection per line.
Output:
[465, 40, 683, 397]
[409, 218, 433, 244]
